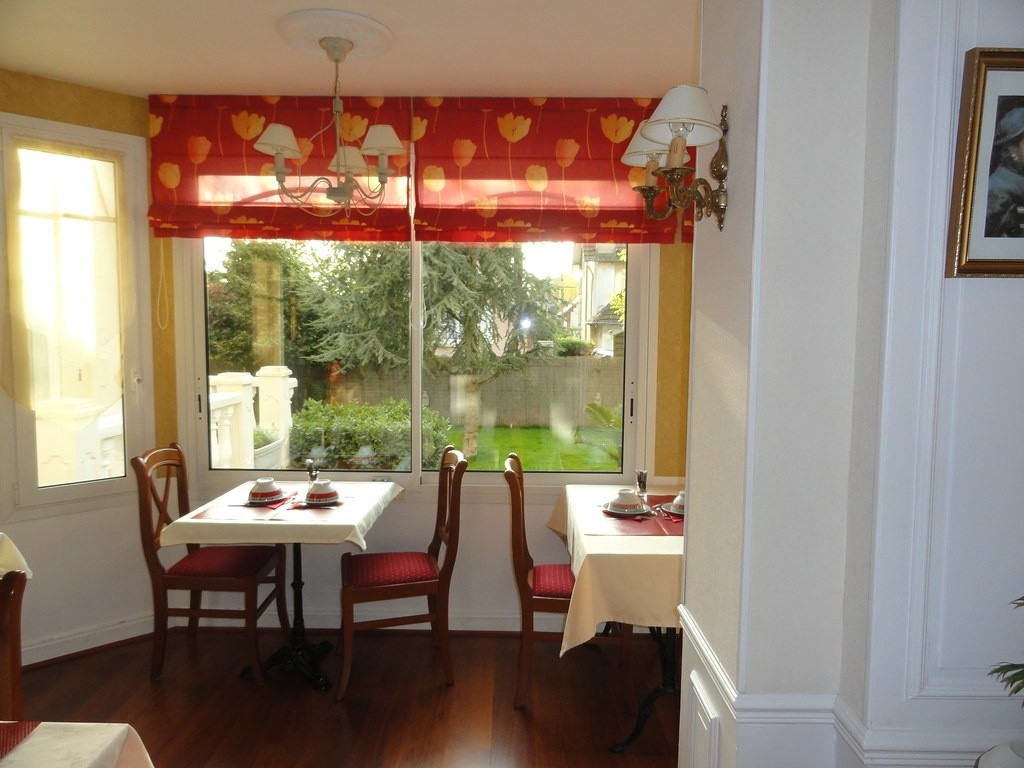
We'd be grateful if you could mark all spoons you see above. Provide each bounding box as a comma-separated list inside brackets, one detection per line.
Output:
[228, 504, 256, 506]
[296, 503, 336, 509]
[656, 508, 664, 517]
[650, 510, 658, 516]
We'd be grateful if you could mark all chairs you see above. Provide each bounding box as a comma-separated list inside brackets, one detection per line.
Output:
[503, 451, 636, 716]
[336, 444, 469, 700]
[131, 442, 293, 686]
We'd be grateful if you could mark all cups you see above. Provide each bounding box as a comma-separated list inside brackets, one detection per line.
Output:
[307, 471, 319, 481]
[637, 482, 647, 491]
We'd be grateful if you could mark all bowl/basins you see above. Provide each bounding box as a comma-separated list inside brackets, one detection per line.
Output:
[353, 446, 380, 461]
[608, 488, 643, 512]
[248, 477, 283, 502]
[309, 447, 327, 461]
[306, 479, 339, 503]
[671, 492, 685, 512]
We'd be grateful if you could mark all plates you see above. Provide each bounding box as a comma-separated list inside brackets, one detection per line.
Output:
[602, 503, 650, 514]
[662, 504, 684, 514]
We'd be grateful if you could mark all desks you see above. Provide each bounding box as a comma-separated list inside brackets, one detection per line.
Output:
[546, 482, 684, 754]
[0, 721, 154, 768]
[159, 478, 406, 693]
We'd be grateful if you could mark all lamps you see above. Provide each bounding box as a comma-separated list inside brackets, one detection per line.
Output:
[620, 84, 728, 231]
[253, 35, 406, 218]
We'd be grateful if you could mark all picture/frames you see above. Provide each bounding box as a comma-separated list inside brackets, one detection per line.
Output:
[943, 47, 1023, 278]
